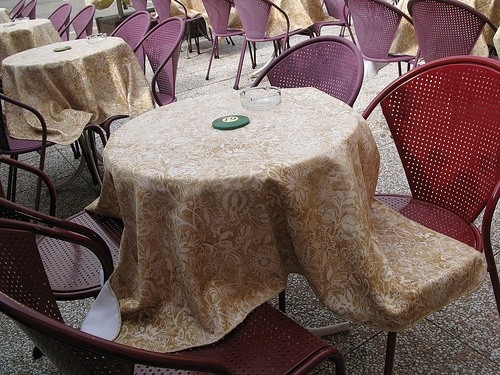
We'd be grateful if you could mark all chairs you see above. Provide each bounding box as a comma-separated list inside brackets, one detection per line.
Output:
[0, 0, 500, 375]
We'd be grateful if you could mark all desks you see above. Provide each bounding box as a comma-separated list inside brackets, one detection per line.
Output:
[81, 87, 487, 354]
[149, 0, 244, 48]
[229, 0, 340, 78]
[363, 0, 500, 138]
[0, 19, 63, 83]
[2, 36, 157, 193]
